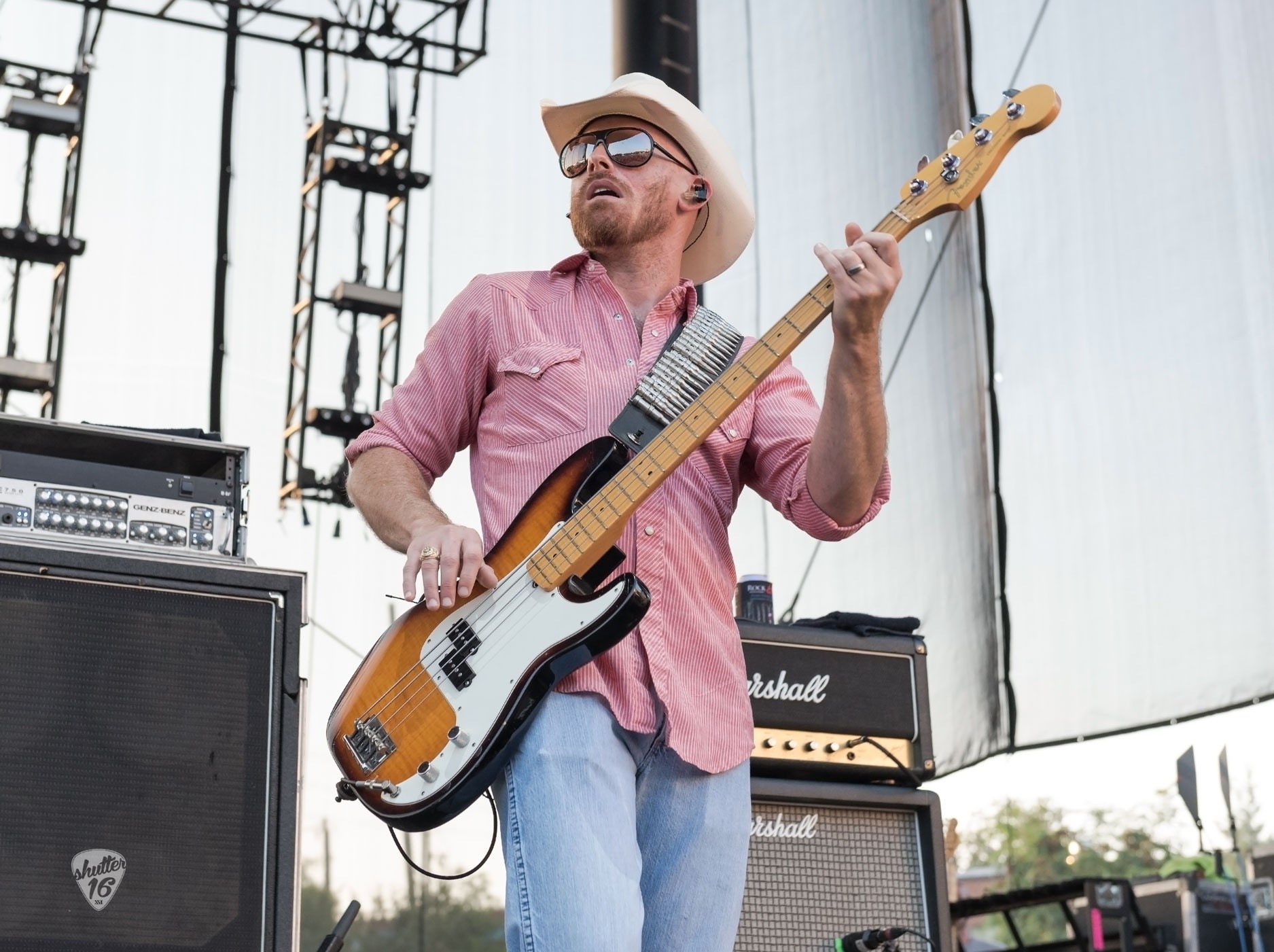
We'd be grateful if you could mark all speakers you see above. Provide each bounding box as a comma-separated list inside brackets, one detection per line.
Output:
[732, 777, 953, 952]
[0, 537, 309, 952]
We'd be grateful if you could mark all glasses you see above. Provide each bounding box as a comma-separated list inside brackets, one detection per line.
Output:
[559, 126, 696, 178]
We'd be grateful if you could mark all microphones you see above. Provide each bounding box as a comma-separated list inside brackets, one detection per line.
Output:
[841, 926, 908, 952]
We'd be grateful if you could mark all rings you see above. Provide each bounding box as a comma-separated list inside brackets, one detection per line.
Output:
[420, 546, 441, 563]
[848, 262, 866, 276]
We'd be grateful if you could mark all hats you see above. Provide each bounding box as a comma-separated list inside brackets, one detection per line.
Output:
[540, 72, 753, 286]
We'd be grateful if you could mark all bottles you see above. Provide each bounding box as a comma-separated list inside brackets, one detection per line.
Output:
[735, 574, 774, 625]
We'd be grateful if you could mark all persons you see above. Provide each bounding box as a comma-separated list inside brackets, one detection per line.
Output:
[342, 70, 904, 952]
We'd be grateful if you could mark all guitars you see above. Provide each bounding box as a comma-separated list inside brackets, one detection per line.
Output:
[324, 81, 1065, 835]
